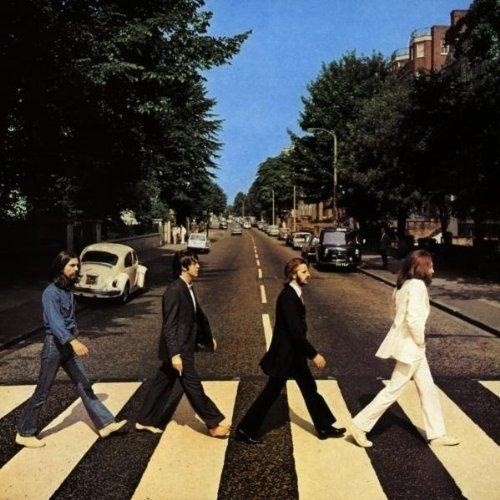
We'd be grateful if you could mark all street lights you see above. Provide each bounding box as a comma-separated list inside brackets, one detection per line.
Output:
[262, 186, 275, 227]
[280, 174, 297, 232]
[307, 127, 338, 225]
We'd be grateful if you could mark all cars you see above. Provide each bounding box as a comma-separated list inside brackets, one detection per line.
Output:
[73, 242, 150, 305]
[219, 214, 291, 243]
[291, 232, 311, 249]
[302, 234, 320, 262]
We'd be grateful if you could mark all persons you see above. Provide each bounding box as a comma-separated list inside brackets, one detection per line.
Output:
[15, 250, 128, 450]
[135, 250, 232, 439]
[179, 224, 187, 244]
[347, 250, 462, 449]
[176, 224, 181, 244]
[236, 258, 347, 444]
[302, 237, 311, 268]
[172, 225, 178, 244]
[379, 227, 389, 271]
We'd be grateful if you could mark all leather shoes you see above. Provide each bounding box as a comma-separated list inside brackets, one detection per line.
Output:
[236, 428, 263, 443]
[319, 426, 346, 439]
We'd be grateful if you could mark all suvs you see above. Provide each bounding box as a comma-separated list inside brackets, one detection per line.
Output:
[315, 226, 366, 271]
[188, 232, 212, 254]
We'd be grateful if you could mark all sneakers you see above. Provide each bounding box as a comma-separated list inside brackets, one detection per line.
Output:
[15, 433, 45, 447]
[99, 419, 128, 437]
[431, 436, 461, 446]
[349, 424, 373, 448]
